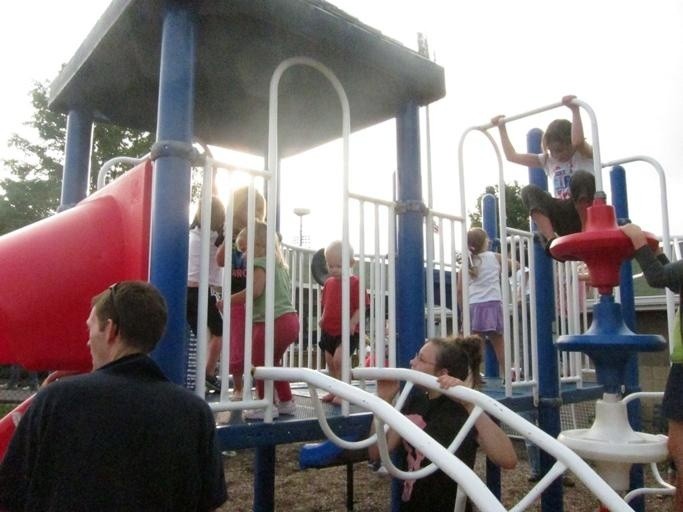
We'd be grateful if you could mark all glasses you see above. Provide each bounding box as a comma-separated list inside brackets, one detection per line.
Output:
[415, 352, 437, 368]
[107, 282, 121, 335]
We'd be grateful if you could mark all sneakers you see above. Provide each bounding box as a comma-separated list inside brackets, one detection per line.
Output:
[545, 237, 564, 263]
[244, 397, 295, 420]
[204, 374, 221, 391]
[320, 392, 341, 405]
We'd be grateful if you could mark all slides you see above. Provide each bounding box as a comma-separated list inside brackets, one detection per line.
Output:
[1, 195, 125, 370]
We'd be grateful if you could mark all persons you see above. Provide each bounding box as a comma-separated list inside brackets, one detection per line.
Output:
[0, 279, 227, 512]
[455, 226, 530, 388]
[319, 239, 369, 405]
[618, 221, 683, 512]
[186, 186, 301, 419]
[491, 96, 594, 264]
[363, 335, 517, 512]
